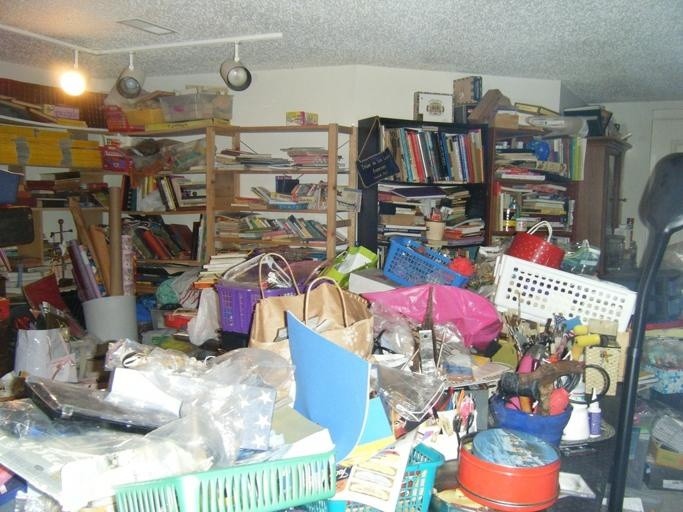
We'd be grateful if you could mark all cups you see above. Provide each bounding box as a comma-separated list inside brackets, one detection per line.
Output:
[425, 219, 447, 240]
[515, 218, 528, 231]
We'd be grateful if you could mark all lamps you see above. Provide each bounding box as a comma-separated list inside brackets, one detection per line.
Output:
[220, 42, 252, 92]
[546, 152, 683, 512]
[116, 51, 145, 99]
[58, 48, 88, 96]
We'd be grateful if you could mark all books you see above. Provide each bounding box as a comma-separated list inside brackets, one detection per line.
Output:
[0, 95, 207, 298]
[378, 125, 486, 246]
[214, 147, 348, 261]
[496, 102, 606, 236]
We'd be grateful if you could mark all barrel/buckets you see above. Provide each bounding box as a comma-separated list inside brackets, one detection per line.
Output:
[506, 218, 567, 270]
[80, 294, 140, 343]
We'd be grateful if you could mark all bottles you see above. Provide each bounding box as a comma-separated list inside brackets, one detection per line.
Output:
[621, 218, 637, 271]
[587, 388, 602, 438]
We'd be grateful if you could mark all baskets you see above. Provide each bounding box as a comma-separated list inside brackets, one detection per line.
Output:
[102, 105, 146, 132]
[584, 345, 622, 397]
[213, 279, 328, 335]
[112, 451, 337, 512]
[489, 253, 639, 335]
[302, 440, 446, 512]
[383, 235, 470, 289]
[506, 231, 566, 269]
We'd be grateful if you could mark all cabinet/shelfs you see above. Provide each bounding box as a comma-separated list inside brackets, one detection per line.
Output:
[359, 116, 489, 269]
[598, 269, 683, 324]
[1, 116, 108, 375]
[118, 123, 358, 296]
[490, 128, 570, 255]
[570, 137, 632, 275]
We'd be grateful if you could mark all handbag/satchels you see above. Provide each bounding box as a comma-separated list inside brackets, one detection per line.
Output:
[353, 147, 401, 187]
[247, 251, 376, 367]
